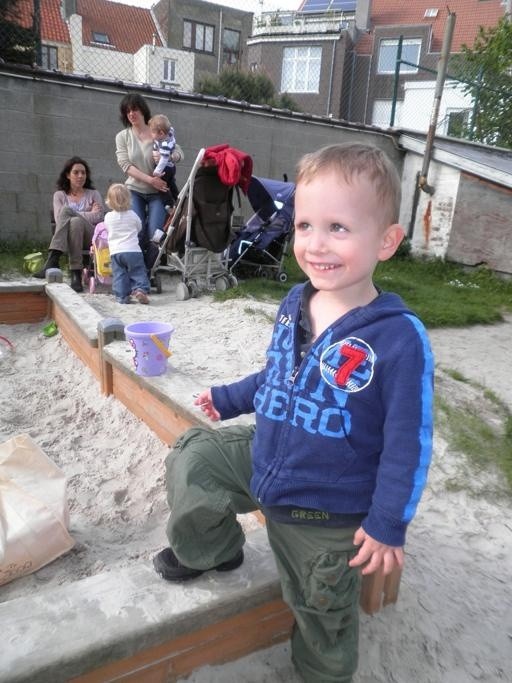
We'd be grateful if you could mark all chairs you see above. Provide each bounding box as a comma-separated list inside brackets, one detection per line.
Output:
[51, 209, 96, 271]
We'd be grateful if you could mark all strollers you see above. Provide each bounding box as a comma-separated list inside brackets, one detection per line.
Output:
[226, 175, 295, 281]
[149, 145, 246, 300]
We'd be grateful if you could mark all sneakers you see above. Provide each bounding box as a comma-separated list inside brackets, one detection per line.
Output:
[153, 548, 243, 580]
[135, 290, 150, 303]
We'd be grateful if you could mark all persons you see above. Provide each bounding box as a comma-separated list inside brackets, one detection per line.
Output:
[113, 93, 184, 285]
[147, 115, 179, 215]
[156, 142, 434, 682]
[33, 157, 104, 294]
[105, 182, 151, 304]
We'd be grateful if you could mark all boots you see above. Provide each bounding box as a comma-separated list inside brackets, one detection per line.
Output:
[71, 270, 83, 292]
[32, 250, 62, 278]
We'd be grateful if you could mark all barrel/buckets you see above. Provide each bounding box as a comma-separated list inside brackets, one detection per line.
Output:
[124, 321, 173, 377]
